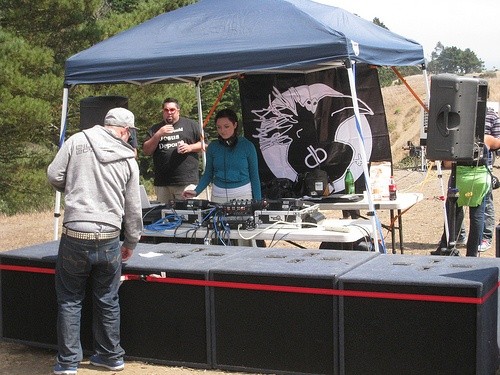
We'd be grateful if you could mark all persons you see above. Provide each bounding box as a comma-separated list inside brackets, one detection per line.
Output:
[430, 79, 500, 257]
[47, 107, 143, 375]
[182, 108, 262, 248]
[142, 96, 204, 201]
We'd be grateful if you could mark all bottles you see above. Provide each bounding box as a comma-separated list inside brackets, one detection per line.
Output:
[389, 176, 397, 200]
[345, 169, 354, 194]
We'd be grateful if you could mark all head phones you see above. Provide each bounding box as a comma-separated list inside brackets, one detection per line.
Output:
[218, 132, 238, 146]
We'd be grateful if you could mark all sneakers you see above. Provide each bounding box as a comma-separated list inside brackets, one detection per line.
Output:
[90, 354, 124, 370]
[53, 364, 77, 374]
[438, 238, 467, 248]
[478, 238, 492, 252]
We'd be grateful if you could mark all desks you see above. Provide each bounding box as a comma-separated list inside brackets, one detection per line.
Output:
[299, 193, 425, 254]
[142, 218, 378, 252]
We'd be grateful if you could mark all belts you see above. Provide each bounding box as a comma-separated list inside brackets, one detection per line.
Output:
[62, 225, 120, 240]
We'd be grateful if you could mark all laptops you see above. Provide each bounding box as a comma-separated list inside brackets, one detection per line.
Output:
[139, 185, 165, 208]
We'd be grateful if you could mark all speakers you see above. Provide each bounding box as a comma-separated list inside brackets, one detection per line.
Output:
[425, 73, 489, 161]
[79, 95, 128, 132]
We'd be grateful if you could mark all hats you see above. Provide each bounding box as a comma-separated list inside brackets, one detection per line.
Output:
[104, 107, 140, 130]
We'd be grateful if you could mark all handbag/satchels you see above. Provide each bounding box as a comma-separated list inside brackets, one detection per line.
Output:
[305, 169, 330, 196]
[491, 175, 500, 190]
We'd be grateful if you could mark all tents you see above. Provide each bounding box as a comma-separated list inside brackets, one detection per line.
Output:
[53, 0, 450, 252]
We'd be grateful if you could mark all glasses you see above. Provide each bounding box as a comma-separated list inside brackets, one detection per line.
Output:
[127, 129, 133, 143]
[163, 108, 178, 114]
